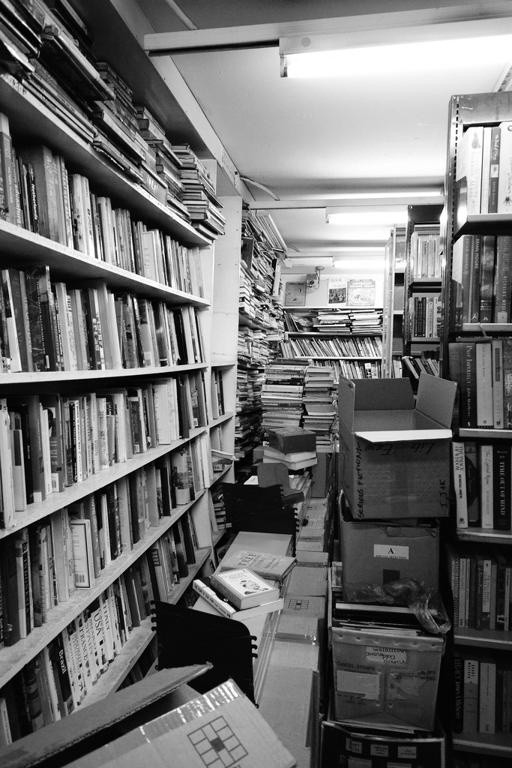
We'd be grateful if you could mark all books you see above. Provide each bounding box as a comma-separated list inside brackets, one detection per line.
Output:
[191, 552, 298, 621]
[1, 0, 226, 748]
[236, 209, 383, 489]
[391, 122, 511, 737]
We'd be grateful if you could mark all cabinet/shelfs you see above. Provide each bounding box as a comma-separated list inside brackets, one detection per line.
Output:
[1, 0, 242, 768]
[446, 84, 512, 767]
[271, 307, 386, 380]
[234, 196, 285, 486]
[378, 223, 406, 378]
[408, 191, 445, 391]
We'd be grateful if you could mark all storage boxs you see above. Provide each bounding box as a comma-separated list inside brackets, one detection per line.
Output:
[330, 488, 441, 610]
[262, 446, 318, 469]
[319, 587, 445, 729]
[0, 660, 299, 767]
[334, 364, 458, 522]
[266, 427, 317, 454]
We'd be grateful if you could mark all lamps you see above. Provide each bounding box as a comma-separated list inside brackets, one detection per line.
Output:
[279, 17, 512, 81]
[324, 205, 409, 226]
